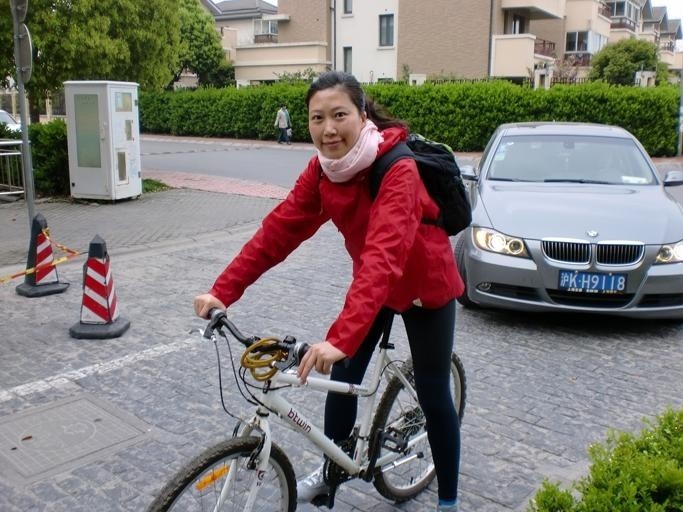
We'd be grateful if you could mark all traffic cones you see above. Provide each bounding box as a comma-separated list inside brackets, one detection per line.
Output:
[12, 214, 69, 298]
[66, 237, 131, 339]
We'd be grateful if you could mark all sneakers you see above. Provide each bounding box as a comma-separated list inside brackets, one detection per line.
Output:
[296, 464, 331, 504]
[436, 503, 458, 512]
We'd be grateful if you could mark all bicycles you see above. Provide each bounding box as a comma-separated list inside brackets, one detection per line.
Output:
[466, 411, 468, 412]
[136, 307, 468, 512]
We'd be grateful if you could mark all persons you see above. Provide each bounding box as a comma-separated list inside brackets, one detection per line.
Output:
[274, 104, 292, 145]
[194, 72, 461, 512]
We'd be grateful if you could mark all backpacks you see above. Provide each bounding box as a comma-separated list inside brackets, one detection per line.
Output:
[368, 134, 472, 235]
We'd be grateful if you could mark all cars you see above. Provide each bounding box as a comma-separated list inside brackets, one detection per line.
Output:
[454, 122, 683, 318]
[0, 110, 21, 132]
